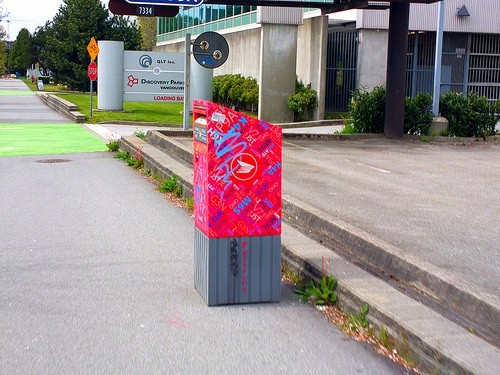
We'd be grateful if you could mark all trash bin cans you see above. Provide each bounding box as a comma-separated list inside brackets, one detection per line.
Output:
[190, 96, 284, 308]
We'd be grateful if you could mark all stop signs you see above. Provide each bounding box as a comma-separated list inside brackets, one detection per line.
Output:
[88, 62, 98, 81]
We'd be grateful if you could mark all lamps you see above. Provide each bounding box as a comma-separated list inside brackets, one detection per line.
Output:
[456, 4, 470, 17]
